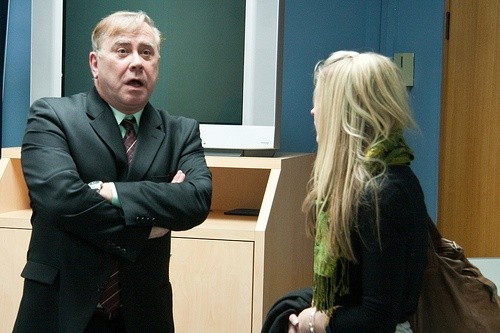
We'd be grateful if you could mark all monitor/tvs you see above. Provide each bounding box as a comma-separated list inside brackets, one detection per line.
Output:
[30, 0, 285, 157]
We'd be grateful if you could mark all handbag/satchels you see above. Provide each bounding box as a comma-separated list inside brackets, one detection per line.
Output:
[408, 238, 500, 333]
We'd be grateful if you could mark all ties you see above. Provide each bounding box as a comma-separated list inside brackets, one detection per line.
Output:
[98, 117, 137, 315]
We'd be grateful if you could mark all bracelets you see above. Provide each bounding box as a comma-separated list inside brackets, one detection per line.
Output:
[308, 310, 317, 333]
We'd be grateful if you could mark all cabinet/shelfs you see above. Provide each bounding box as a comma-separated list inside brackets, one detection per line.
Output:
[0, 146, 317, 333]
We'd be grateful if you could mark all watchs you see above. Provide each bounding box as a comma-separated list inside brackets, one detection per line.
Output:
[88, 180, 104, 193]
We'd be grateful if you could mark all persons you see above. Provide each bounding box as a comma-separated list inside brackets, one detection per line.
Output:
[288, 51, 441, 333]
[11, 11, 213, 333]
